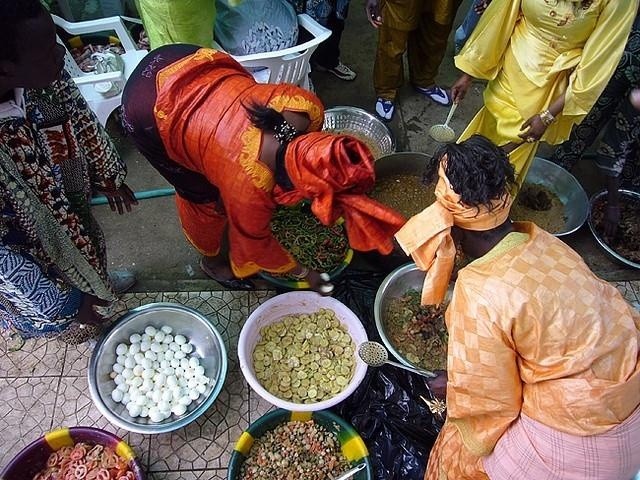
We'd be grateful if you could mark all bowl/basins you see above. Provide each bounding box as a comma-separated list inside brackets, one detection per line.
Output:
[4, 428, 142, 480]
[585, 190, 638, 270]
[236, 292, 372, 412]
[86, 303, 228, 439]
[257, 200, 356, 290]
[224, 407, 373, 480]
[373, 264, 453, 382]
[368, 151, 442, 223]
[507, 156, 593, 239]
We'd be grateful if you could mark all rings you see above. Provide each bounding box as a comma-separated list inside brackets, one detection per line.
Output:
[527, 136, 534, 143]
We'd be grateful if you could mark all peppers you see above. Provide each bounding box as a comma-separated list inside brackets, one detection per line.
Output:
[260, 201, 349, 280]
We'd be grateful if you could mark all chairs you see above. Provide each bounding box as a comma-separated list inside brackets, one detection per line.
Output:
[212, 13, 335, 92]
[50, 14, 147, 129]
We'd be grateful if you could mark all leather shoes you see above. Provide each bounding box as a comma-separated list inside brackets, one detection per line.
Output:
[197, 256, 259, 292]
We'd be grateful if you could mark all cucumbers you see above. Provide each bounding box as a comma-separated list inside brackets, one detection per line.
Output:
[252, 307, 356, 404]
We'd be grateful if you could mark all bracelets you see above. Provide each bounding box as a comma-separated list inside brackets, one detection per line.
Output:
[608, 207, 617, 210]
[540, 110, 556, 127]
[292, 269, 309, 278]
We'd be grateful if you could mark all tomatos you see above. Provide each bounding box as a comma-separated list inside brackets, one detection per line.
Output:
[34, 442, 135, 480]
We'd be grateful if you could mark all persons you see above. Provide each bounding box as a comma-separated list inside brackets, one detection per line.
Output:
[450, 1, 639, 199]
[548, 1, 639, 237]
[291, 0, 356, 81]
[393, 134, 639, 479]
[454, 0, 491, 55]
[0, 0, 140, 345]
[120, 44, 408, 290]
[365, 0, 462, 123]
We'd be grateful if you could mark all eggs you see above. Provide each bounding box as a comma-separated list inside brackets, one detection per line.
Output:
[109, 325, 210, 422]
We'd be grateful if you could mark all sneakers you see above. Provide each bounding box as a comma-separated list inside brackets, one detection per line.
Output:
[313, 57, 358, 83]
[410, 79, 453, 108]
[373, 88, 399, 123]
[107, 269, 140, 296]
